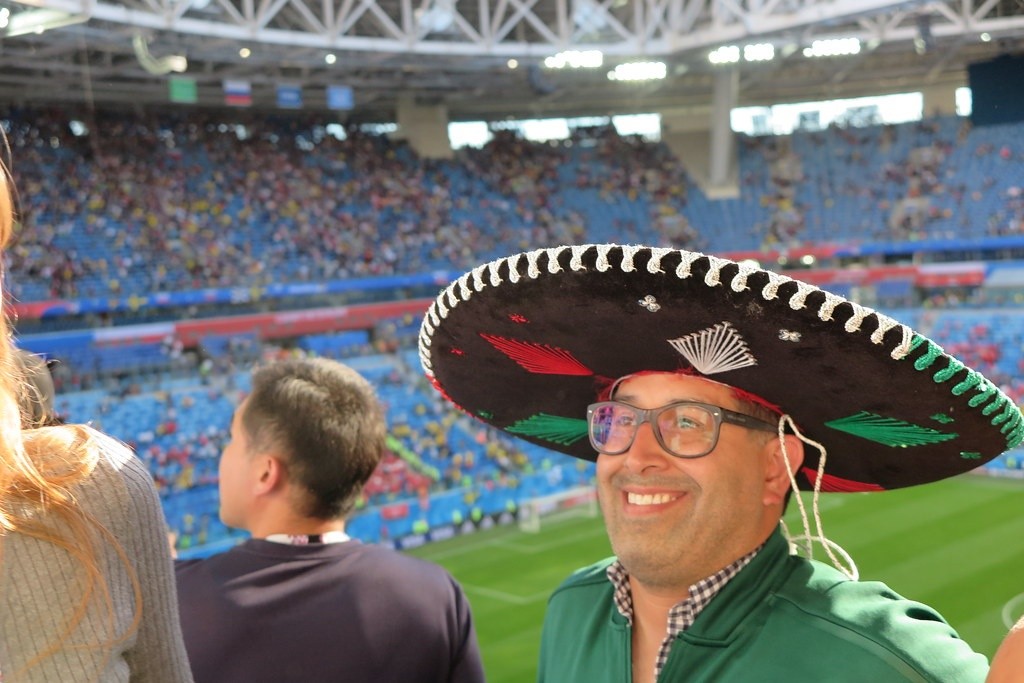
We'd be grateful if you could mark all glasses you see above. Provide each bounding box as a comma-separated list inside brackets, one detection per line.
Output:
[586, 401, 779, 458]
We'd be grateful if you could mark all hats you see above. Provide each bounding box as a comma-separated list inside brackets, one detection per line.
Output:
[418, 243, 1024, 492]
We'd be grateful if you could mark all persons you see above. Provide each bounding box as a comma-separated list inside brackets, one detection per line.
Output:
[168, 354, 488, 683]
[1, 157, 193, 683]
[0, 104, 1024, 562]
[418, 242, 1024, 683]
